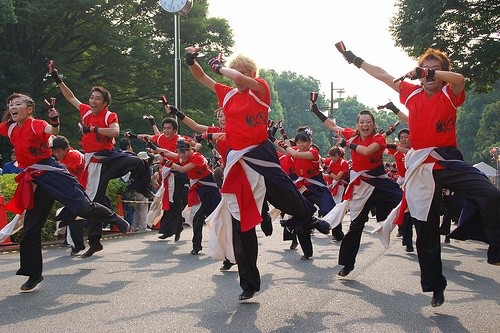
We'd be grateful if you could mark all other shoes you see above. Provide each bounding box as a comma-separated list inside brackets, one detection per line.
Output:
[239, 290, 256, 301]
[338, 265, 354, 275]
[116, 215, 130, 233]
[81, 241, 103, 258]
[315, 219, 331, 235]
[220, 258, 233, 270]
[432, 280, 447, 307]
[70, 244, 85, 256]
[20, 276, 45, 291]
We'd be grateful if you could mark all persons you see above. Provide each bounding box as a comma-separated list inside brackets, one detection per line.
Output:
[343, 47, 500, 307]
[52, 136, 112, 255]
[0, 92, 130, 292]
[310, 100, 405, 277]
[48, 60, 156, 258]
[2, 148, 23, 174]
[182, 46, 332, 300]
[115, 101, 461, 271]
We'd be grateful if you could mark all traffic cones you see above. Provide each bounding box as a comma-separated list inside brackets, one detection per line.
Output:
[110, 195, 126, 233]
[0, 195, 19, 246]
